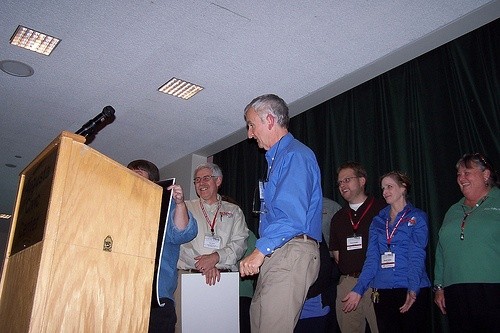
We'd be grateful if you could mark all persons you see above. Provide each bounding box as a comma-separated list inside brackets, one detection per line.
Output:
[221, 94, 342, 333]
[342, 171, 434, 333]
[125, 159, 198, 333]
[329, 161, 388, 333]
[433, 150, 500, 332]
[174, 163, 250, 333]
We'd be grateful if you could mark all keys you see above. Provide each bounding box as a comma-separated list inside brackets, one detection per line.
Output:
[371, 288, 380, 303]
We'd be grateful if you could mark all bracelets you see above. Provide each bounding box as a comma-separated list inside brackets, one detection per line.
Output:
[433, 283, 444, 290]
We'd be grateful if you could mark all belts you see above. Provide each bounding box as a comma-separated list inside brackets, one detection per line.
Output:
[296, 235, 318, 244]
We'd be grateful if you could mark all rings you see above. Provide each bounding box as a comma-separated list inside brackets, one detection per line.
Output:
[201, 268, 205, 271]
[249, 271, 254, 274]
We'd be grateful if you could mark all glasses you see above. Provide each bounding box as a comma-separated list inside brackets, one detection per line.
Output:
[338, 177, 358, 186]
[194, 176, 215, 183]
[252, 187, 261, 213]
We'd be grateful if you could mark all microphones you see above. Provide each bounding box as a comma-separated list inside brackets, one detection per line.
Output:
[75, 105, 115, 137]
